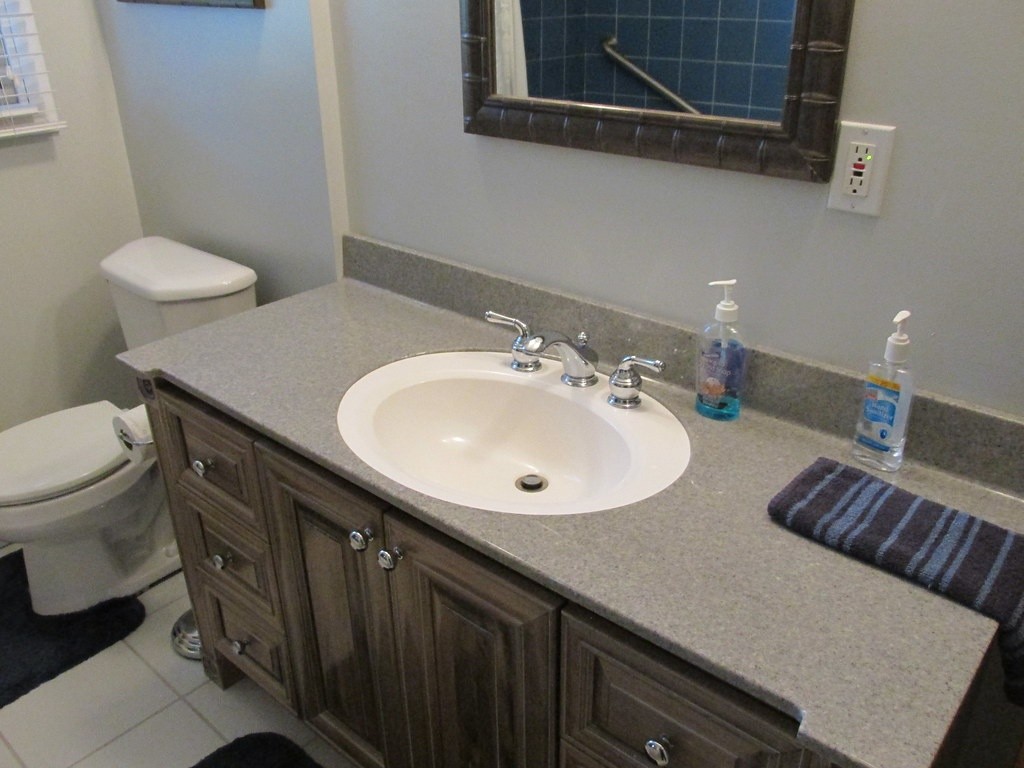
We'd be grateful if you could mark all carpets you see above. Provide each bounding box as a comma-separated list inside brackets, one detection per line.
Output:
[192, 731, 324, 767]
[0, 547, 146, 707]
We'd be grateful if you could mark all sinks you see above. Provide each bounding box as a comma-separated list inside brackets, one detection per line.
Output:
[335, 351, 692, 516]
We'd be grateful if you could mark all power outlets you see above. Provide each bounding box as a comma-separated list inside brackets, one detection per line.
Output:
[828, 120, 897, 216]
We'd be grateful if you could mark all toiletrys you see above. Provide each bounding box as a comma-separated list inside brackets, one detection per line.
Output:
[849, 309, 915, 473]
[695, 278, 747, 421]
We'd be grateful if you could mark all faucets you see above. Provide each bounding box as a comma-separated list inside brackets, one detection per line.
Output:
[512, 328, 600, 388]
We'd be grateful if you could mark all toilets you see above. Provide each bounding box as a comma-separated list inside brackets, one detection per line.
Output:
[0, 237, 258, 616]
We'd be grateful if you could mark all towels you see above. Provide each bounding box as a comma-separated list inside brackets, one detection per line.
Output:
[766, 455, 1024, 624]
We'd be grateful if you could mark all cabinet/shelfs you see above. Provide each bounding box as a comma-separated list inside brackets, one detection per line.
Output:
[131, 376, 848, 768]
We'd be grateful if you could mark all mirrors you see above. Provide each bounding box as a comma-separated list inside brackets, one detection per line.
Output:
[459, 0, 857, 185]
